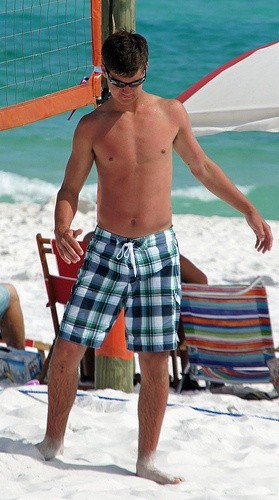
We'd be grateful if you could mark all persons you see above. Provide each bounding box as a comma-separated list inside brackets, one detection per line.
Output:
[0, 283, 27, 350]
[82, 231, 208, 376]
[34, 27, 275, 487]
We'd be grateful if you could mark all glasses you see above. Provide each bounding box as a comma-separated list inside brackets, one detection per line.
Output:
[102, 61, 146, 88]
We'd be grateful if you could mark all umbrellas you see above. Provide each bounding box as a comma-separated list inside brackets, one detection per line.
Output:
[175, 39, 279, 137]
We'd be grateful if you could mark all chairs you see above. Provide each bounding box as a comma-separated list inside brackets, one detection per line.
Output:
[35, 229, 279, 401]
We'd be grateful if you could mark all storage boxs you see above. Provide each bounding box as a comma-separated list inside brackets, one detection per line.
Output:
[0, 345, 44, 385]
